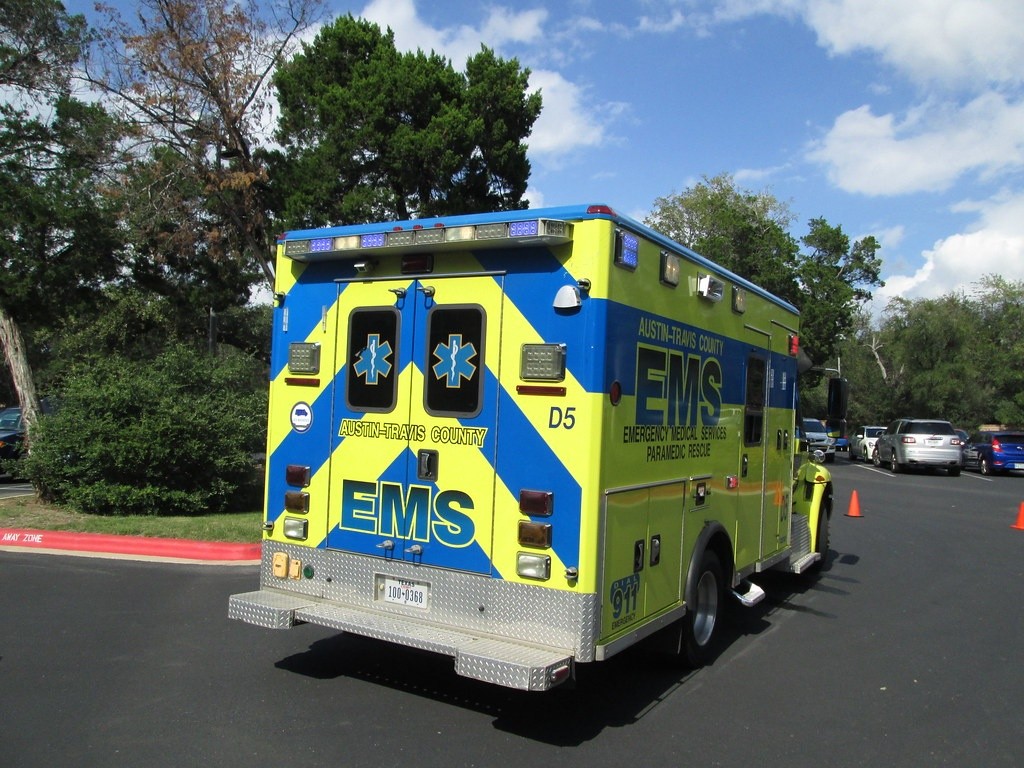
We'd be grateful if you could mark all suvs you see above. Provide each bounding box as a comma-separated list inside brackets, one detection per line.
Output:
[803, 418, 835, 462]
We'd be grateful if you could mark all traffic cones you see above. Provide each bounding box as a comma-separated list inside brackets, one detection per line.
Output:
[1009, 501, 1024, 529]
[844, 488, 864, 518]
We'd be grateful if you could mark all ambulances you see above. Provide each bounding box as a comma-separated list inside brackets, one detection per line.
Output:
[229, 200, 833, 692]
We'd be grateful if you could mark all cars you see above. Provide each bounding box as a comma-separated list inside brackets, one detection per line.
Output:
[953, 429, 969, 442]
[962, 430, 1024, 476]
[836, 438, 848, 452]
[847, 425, 886, 463]
[871, 418, 962, 476]
[0, 398, 55, 482]
